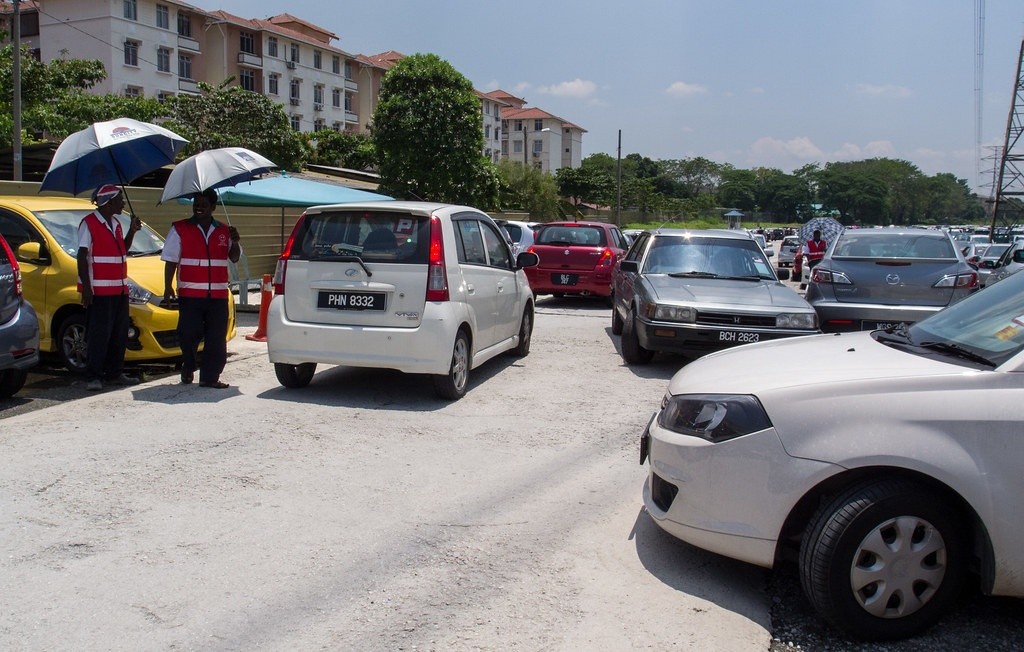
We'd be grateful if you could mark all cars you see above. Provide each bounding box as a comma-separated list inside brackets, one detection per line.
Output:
[524, 220, 630, 308]
[493, 219, 543, 253]
[981, 239, 1024, 287]
[803, 228, 981, 334]
[640, 268, 1024, 643]
[0, 194, 237, 377]
[0, 234, 41, 400]
[608, 227, 824, 366]
[265, 199, 539, 400]
[622, 222, 1024, 271]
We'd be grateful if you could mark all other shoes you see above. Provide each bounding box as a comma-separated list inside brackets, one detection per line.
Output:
[112, 375, 139, 384]
[181, 372, 194, 384]
[199, 381, 229, 388]
[85, 381, 102, 390]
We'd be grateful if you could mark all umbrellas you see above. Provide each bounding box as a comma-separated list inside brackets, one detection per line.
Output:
[157, 147, 278, 229]
[177, 170, 396, 253]
[38, 118, 191, 217]
[798, 217, 845, 249]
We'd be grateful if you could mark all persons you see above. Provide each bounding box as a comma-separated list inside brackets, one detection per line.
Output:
[160, 188, 240, 389]
[77, 184, 142, 392]
[756, 227, 769, 242]
[803, 230, 828, 272]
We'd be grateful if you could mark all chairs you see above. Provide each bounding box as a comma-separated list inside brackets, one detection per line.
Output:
[363, 228, 397, 251]
[227, 253, 263, 307]
[713, 248, 751, 276]
[849, 240, 871, 256]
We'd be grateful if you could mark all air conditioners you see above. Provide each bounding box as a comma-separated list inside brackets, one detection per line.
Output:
[291, 61, 298, 69]
[318, 104, 325, 111]
